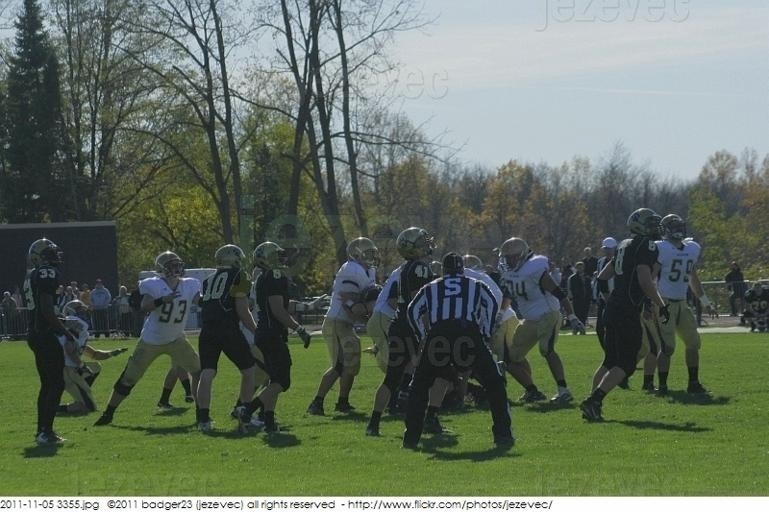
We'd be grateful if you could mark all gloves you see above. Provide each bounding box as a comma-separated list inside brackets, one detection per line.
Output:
[299, 328, 311, 349]
[161, 291, 181, 303]
[705, 305, 718, 320]
[659, 303, 671, 325]
[81, 363, 95, 377]
[568, 318, 586, 336]
[112, 347, 129, 356]
[313, 293, 330, 311]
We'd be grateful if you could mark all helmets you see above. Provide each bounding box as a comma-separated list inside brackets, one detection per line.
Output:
[215, 244, 246, 270]
[626, 208, 687, 241]
[361, 283, 382, 301]
[155, 252, 184, 280]
[253, 241, 289, 270]
[346, 237, 380, 268]
[498, 238, 530, 274]
[29, 239, 64, 268]
[61, 299, 91, 322]
[396, 227, 438, 261]
[462, 255, 483, 270]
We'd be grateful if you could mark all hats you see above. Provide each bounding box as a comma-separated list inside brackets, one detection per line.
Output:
[601, 237, 617, 249]
[443, 253, 464, 269]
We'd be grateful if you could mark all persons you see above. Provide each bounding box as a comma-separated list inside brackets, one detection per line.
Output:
[2, 208, 767, 451]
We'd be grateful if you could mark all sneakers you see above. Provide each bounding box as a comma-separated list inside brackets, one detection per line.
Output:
[307, 400, 325, 416]
[195, 409, 282, 434]
[516, 391, 603, 423]
[184, 394, 194, 402]
[93, 411, 113, 426]
[36, 429, 68, 445]
[334, 403, 367, 416]
[156, 402, 177, 412]
[491, 425, 515, 446]
[687, 383, 713, 397]
[365, 425, 384, 438]
[617, 378, 668, 396]
[421, 418, 453, 433]
